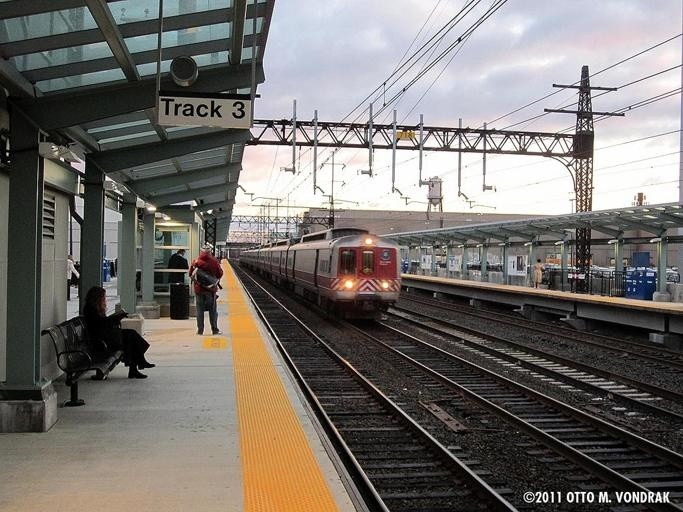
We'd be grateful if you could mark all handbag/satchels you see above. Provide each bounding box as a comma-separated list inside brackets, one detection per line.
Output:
[190, 267, 222, 292]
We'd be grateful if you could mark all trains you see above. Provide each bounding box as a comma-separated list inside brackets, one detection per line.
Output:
[239, 226, 402, 320]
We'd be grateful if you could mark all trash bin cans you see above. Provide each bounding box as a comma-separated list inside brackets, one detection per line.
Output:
[103, 259, 111, 282]
[170, 283, 189, 320]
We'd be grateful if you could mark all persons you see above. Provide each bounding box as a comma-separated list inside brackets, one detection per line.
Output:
[529, 257, 545, 290]
[66, 257, 80, 301]
[188, 241, 224, 336]
[80, 285, 156, 380]
[165, 249, 189, 284]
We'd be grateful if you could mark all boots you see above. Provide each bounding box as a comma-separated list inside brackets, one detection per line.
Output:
[128, 368, 147, 378]
[138, 359, 154, 369]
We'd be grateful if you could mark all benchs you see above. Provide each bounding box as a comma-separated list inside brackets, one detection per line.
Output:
[41, 315, 124, 407]
